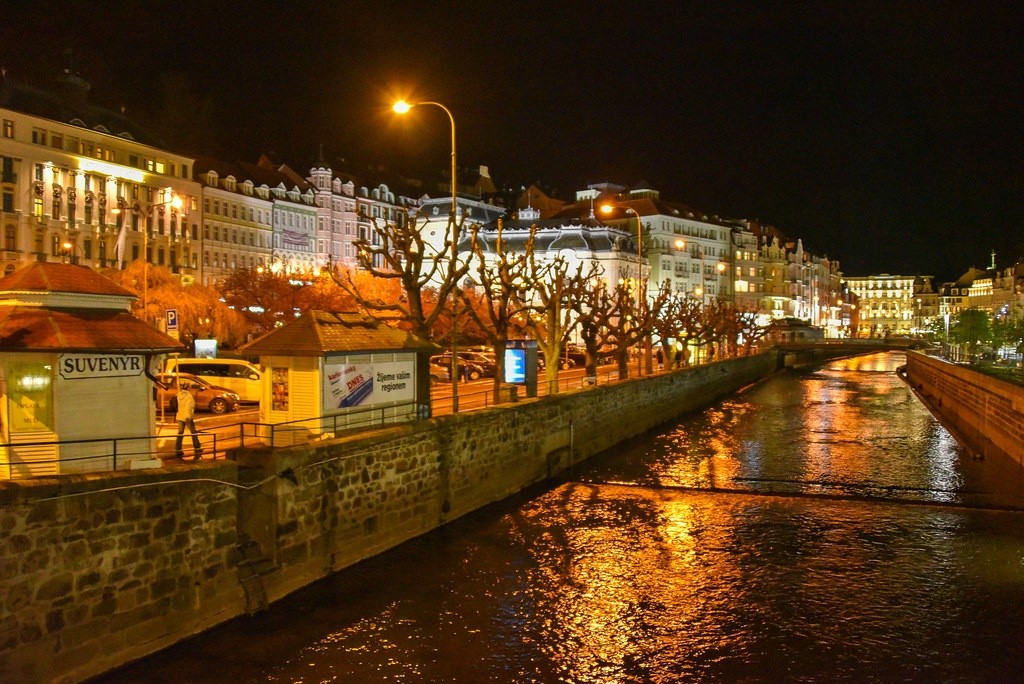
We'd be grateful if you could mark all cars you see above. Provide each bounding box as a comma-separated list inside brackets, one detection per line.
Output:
[630, 345, 658, 357]
[562, 346, 586, 366]
[536, 351, 575, 369]
[536, 357, 545, 371]
[430, 356, 485, 381]
[153, 373, 240, 415]
[429, 362, 450, 386]
[444, 345, 497, 379]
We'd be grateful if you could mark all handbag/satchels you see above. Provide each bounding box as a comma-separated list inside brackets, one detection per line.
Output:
[169, 395, 179, 413]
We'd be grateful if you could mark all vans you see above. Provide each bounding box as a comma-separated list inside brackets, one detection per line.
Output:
[160, 358, 264, 404]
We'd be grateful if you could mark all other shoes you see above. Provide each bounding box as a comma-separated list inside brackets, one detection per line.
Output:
[193, 447, 203, 461]
[175, 450, 184, 458]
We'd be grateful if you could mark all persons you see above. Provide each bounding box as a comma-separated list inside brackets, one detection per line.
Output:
[174, 381, 204, 455]
[675, 350, 681, 368]
[656, 348, 664, 370]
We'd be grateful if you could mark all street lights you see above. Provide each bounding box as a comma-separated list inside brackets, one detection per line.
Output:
[393, 98, 460, 412]
[676, 239, 705, 314]
[111, 196, 181, 324]
[601, 204, 643, 378]
